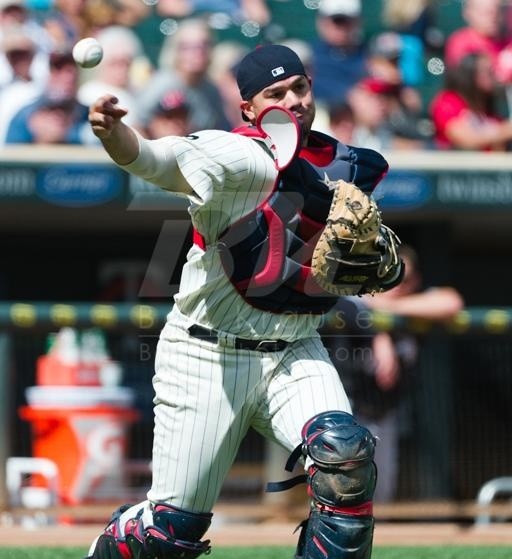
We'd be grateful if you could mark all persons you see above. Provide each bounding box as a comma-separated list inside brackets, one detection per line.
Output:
[257, 291, 364, 511]
[0, 1, 512, 155]
[344, 243, 463, 502]
[85, 44, 407, 557]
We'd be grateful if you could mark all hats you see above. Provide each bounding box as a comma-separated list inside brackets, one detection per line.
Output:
[236, 45, 308, 101]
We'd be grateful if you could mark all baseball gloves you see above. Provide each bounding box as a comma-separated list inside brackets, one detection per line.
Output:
[311, 179, 402, 296]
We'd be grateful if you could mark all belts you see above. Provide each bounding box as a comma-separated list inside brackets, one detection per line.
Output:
[188, 324, 287, 353]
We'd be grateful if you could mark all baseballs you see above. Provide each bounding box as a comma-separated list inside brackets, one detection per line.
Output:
[72, 37, 102, 67]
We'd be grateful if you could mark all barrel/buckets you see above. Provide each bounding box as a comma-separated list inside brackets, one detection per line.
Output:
[19, 385, 142, 525]
